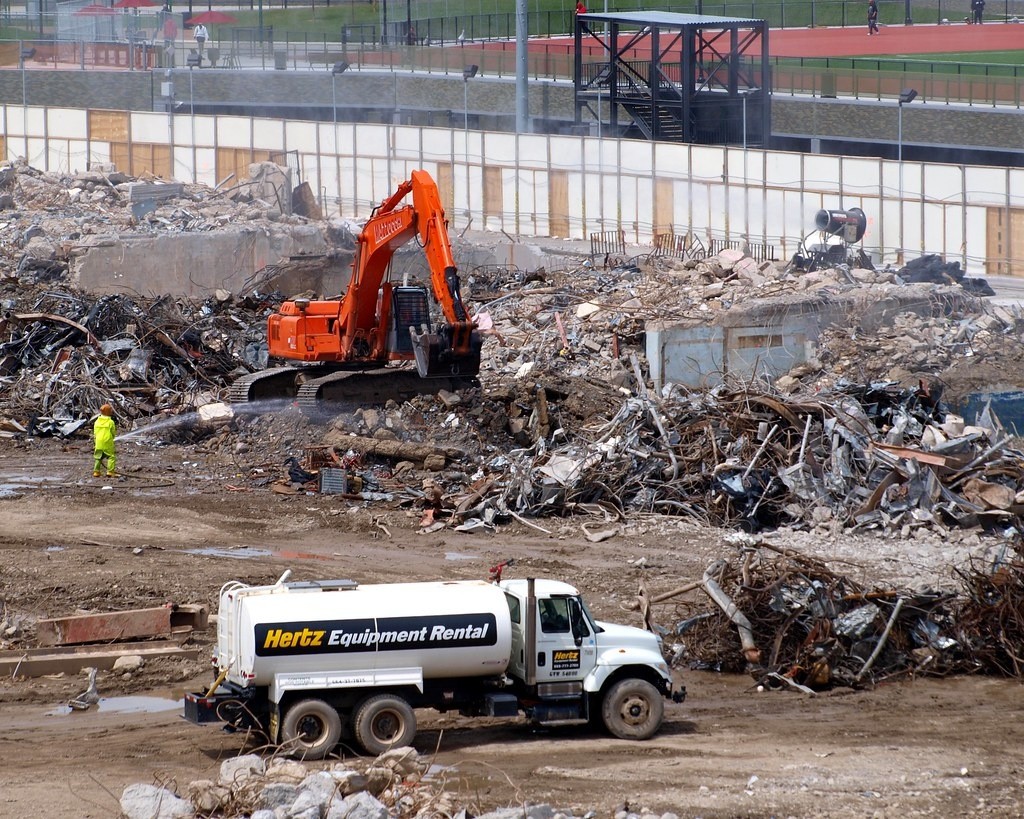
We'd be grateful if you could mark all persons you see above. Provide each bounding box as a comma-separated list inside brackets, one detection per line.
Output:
[405, 27, 417, 63]
[971, 0, 985, 25]
[93, 404, 116, 476]
[194, 23, 209, 53]
[575, 2, 589, 36]
[164, 17, 178, 47]
[867, 0, 879, 35]
[159, 4, 169, 30]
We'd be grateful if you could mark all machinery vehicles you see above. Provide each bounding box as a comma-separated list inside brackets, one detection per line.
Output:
[232, 169, 484, 402]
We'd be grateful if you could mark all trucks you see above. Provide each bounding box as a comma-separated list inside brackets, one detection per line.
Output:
[183, 581, 686, 759]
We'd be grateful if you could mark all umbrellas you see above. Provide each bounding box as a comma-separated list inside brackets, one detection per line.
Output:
[111, 0, 156, 8]
[185, 11, 239, 47]
[72, 4, 121, 17]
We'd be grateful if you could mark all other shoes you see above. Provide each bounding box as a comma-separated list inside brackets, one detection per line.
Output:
[107, 472, 115, 476]
[93, 472, 101, 476]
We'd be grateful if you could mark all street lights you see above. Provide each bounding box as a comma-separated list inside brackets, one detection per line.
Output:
[332, 61, 348, 122]
[598, 70, 614, 138]
[899, 87, 917, 160]
[21, 47, 36, 104]
[743, 88, 764, 149]
[187, 54, 203, 114]
[463, 65, 478, 129]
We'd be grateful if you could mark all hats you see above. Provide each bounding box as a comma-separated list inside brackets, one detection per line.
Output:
[100, 404, 112, 415]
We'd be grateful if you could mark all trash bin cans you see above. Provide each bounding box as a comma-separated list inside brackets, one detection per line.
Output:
[821, 73, 837, 99]
